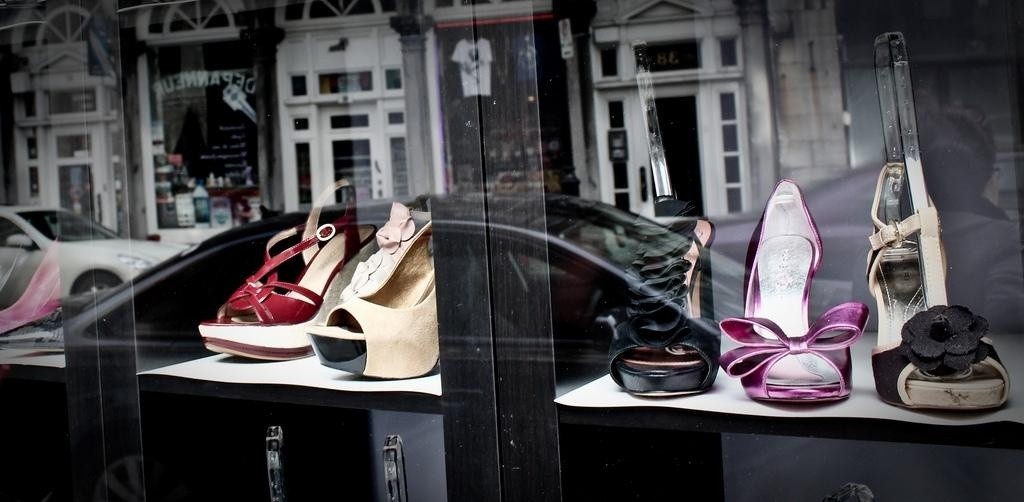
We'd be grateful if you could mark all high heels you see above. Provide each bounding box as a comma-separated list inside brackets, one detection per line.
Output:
[306, 202, 441, 380]
[199, 179, 378, 361]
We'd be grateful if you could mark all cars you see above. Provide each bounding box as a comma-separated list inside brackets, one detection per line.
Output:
[670, 158, 1024, 334]
[2, 191, 714, 502]
[0, 201, 194, 318]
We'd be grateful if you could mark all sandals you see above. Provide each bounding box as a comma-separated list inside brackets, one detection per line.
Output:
[866, 163, 1010, 410]
[719, 179, 870, 402]
[608, 201, 721, 396]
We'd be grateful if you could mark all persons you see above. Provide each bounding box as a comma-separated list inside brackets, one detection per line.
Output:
[434, 122, 599, 500]
